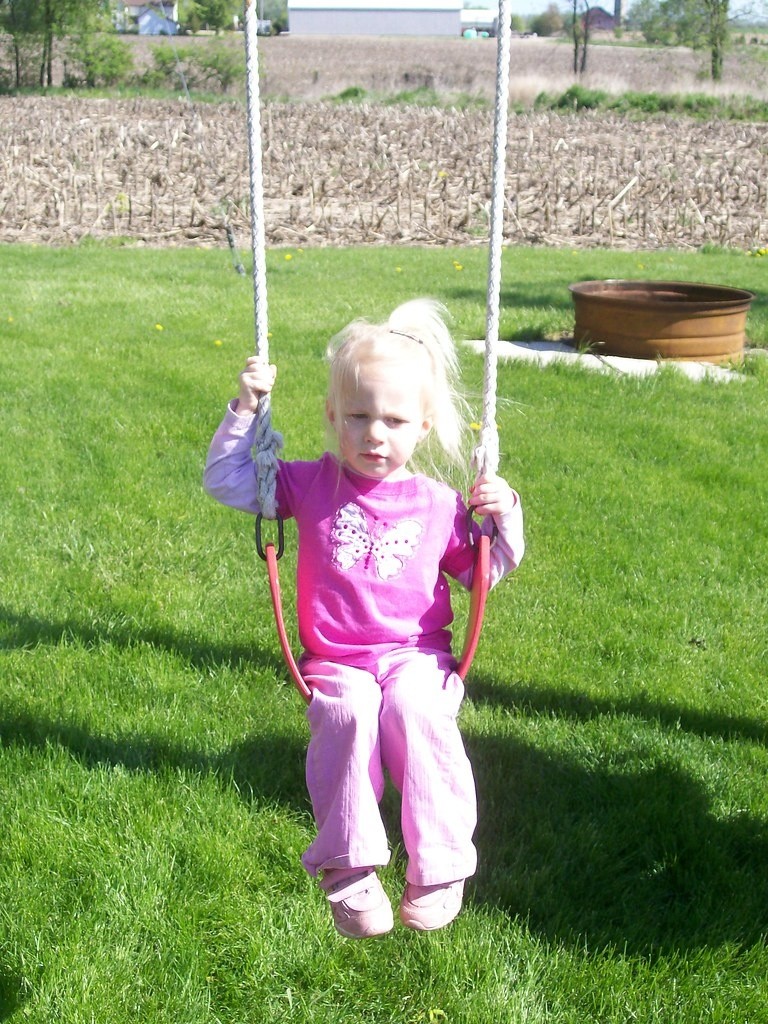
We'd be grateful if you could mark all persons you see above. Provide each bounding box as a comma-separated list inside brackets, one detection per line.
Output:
[204, 298, 525, 939]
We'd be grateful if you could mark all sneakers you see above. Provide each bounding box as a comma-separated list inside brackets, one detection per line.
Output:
[400, 879, 464, 931]
[318, 862, 394, 939]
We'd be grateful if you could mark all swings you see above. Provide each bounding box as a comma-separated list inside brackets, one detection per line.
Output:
[242, 2, 510, 724]
